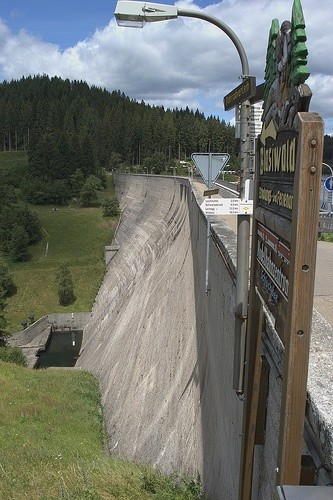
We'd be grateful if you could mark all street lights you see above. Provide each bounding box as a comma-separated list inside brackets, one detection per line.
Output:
[113, 0, 257, 401]
